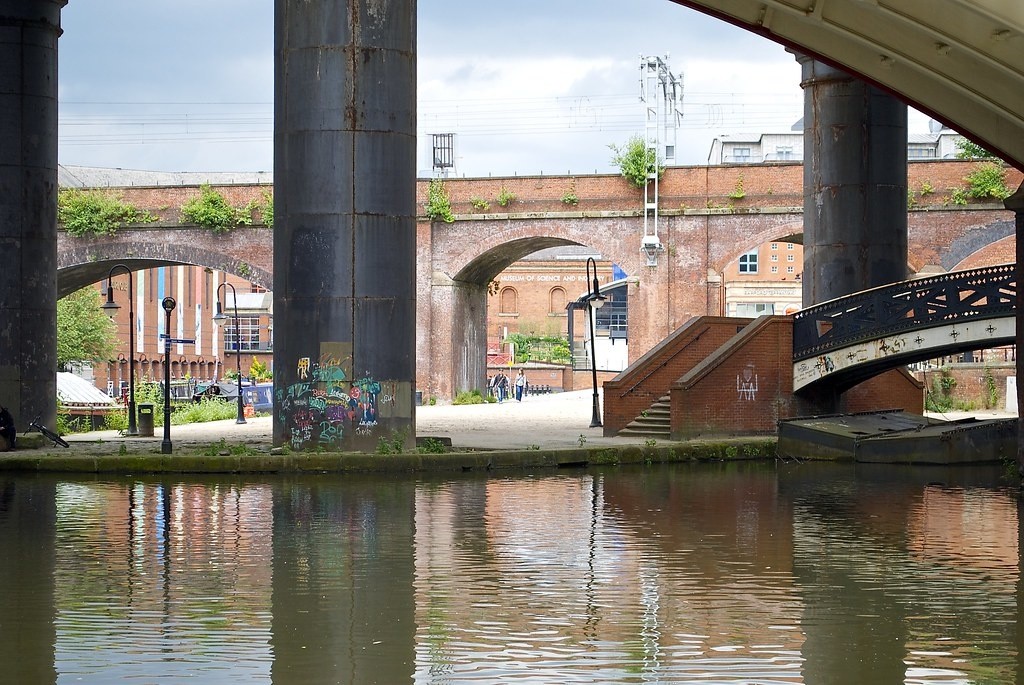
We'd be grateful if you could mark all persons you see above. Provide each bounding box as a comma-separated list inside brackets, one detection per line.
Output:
[488, 369, 510, 404]
[0, 404, 17, 453]
[513, 368, 527, 403]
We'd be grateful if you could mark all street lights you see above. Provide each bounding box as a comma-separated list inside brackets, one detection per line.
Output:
[100, 265, 140, 436]
[586, 257, 607, 428]
[162, 297, 176, 455]
[211, 282, 247, 424]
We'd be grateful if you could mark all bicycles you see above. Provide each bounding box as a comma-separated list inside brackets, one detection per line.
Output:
[23, 410, 69, 448]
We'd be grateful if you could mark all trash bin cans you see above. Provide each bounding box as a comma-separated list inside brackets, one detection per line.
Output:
[138, 402, 154, 436]
[416, 391, 423, 406]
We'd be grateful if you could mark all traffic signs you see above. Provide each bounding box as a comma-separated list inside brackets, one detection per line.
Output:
[160, 334, 196, 344]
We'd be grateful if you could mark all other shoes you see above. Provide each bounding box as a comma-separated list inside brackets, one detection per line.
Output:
[499, 400, 502, 404]
[517, 400, 521, 403]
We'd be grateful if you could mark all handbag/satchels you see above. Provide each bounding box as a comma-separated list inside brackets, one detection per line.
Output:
[494, 384, 498, 391]
